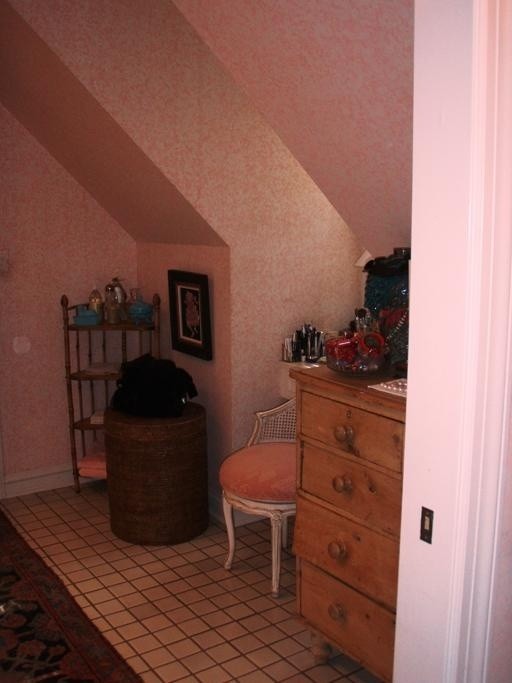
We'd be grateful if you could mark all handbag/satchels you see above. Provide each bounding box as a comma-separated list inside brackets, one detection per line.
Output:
[110, 355, 200, 419]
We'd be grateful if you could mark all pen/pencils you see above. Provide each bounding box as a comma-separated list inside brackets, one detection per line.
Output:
[301, 322, 320, 360]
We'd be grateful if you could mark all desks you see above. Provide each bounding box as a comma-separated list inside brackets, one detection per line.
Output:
[101, 402, 209, 543]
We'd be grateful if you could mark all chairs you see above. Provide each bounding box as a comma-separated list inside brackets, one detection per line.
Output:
[219, 395, 298, 599]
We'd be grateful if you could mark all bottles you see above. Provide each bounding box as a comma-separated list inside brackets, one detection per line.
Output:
[88, 277, 128, 325]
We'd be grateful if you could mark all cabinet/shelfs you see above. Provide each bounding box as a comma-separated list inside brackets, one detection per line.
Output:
[59, 293, 163, 496]
[288, 367, 406, 683]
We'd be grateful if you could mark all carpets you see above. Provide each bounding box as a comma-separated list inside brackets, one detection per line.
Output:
[0, 505, 145, 683]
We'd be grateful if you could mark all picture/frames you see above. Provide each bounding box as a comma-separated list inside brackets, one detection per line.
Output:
[167, 268, 212, 362]
